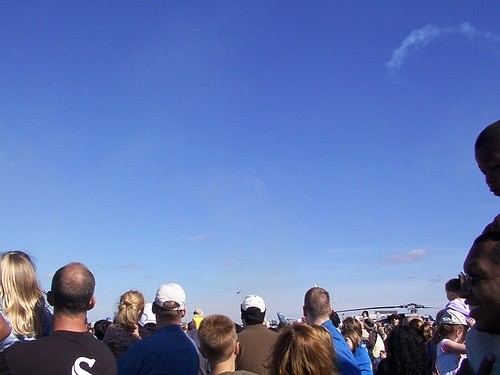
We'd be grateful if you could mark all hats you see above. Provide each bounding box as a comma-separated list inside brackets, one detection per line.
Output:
[241, 295, 266, 313]
[438, 308, 469, 326]
[154, 283, 186, 311]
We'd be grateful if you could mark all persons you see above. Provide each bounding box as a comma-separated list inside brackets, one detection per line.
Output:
[88, 278, 475, 375]
[464, 119, 500, 375]
[458, 232, 500, 375]
[0, 262, 120, 375]
[0, 251, 56, 350]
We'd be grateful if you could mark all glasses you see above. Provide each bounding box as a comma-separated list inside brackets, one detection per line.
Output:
[457, 271, 500, 285]
